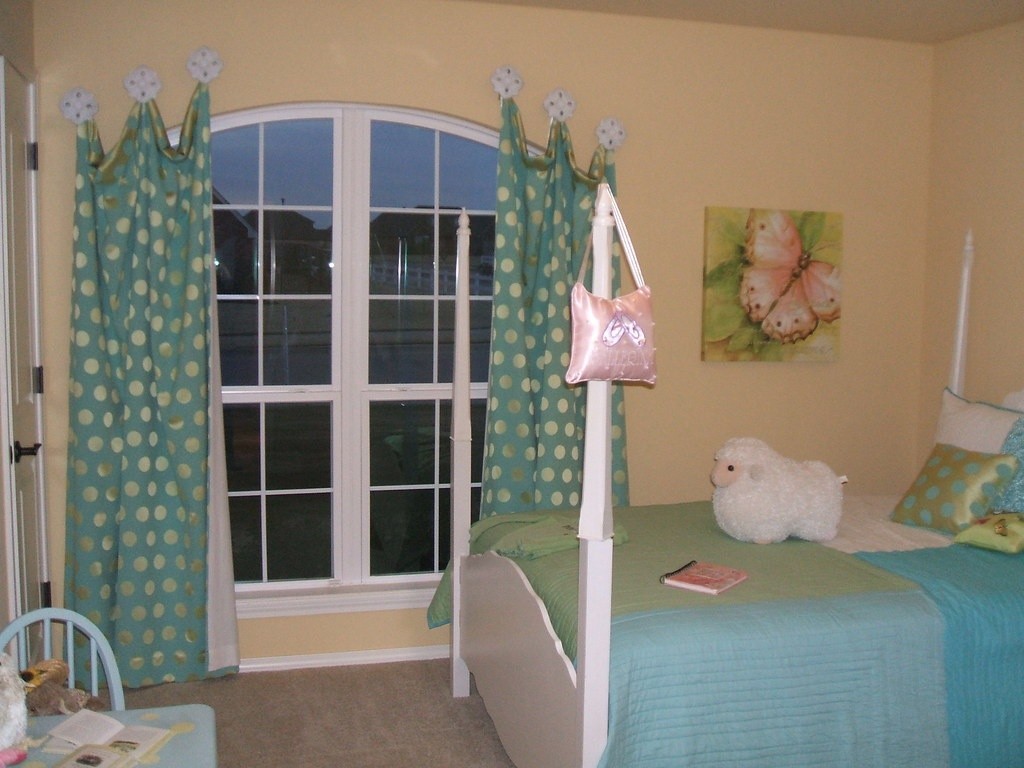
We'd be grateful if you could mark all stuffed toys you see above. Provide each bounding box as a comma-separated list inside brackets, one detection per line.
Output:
[0, 652, 27, 768]
[26, 679, 111, 716]
[711, 437, 848, 544]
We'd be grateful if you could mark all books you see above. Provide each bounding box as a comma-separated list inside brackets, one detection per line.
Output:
[41, 708, 176, 768]
[660, 559, 747, 595]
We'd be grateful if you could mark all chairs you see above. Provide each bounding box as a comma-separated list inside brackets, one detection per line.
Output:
[1, 607, 125, 711]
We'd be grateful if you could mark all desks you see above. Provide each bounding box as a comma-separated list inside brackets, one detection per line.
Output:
[0, 704, 217, 768]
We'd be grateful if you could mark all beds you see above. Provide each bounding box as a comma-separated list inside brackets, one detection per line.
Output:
[425, 176, 1024, 768]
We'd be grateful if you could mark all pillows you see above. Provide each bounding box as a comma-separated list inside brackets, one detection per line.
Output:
[934, 386, 1024, 514]
[887, 441, 1020, 531]
[953, 511, 1024, 556]
